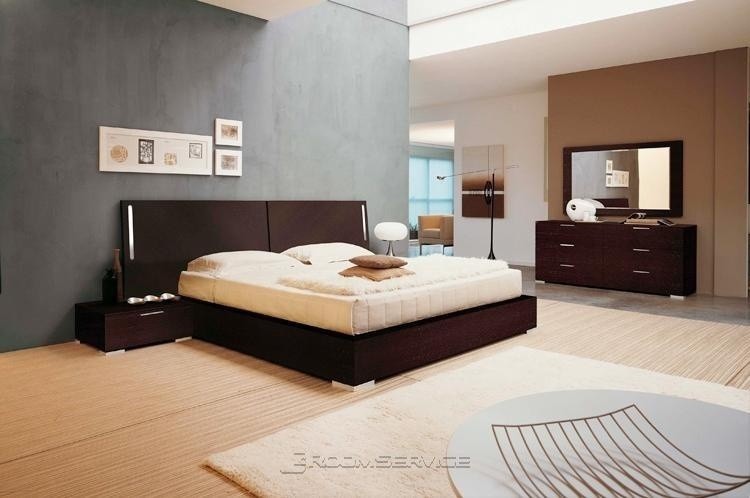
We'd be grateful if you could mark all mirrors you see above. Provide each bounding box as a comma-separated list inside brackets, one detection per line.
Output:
[562, 140, 682, 217]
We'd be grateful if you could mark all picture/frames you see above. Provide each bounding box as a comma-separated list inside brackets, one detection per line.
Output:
[98, 118, 243, 177]
[605, 160, 629, 187]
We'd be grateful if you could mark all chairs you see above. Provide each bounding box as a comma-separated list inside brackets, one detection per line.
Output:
[418, 214, 453, 245]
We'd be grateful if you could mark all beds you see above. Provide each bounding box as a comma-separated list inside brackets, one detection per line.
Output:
[121, 200, 537, 391]
[593, 198, 629, 208]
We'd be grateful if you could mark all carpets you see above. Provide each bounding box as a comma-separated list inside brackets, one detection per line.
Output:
[199, 346, 750, 497]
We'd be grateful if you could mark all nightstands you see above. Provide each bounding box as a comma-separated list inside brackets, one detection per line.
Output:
[74, 300, 194, 356]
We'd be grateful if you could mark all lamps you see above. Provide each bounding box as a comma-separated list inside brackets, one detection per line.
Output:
[437, 165, 495, 260]
[374, 221, 408, 257]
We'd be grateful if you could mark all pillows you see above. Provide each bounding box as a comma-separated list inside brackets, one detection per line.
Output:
[184, 241, 415, 282]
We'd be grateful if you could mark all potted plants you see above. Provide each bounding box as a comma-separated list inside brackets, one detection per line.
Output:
[408, 222, 418, 239]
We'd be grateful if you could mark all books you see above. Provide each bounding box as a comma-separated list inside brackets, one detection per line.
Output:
[621, 212, 675, 227]
